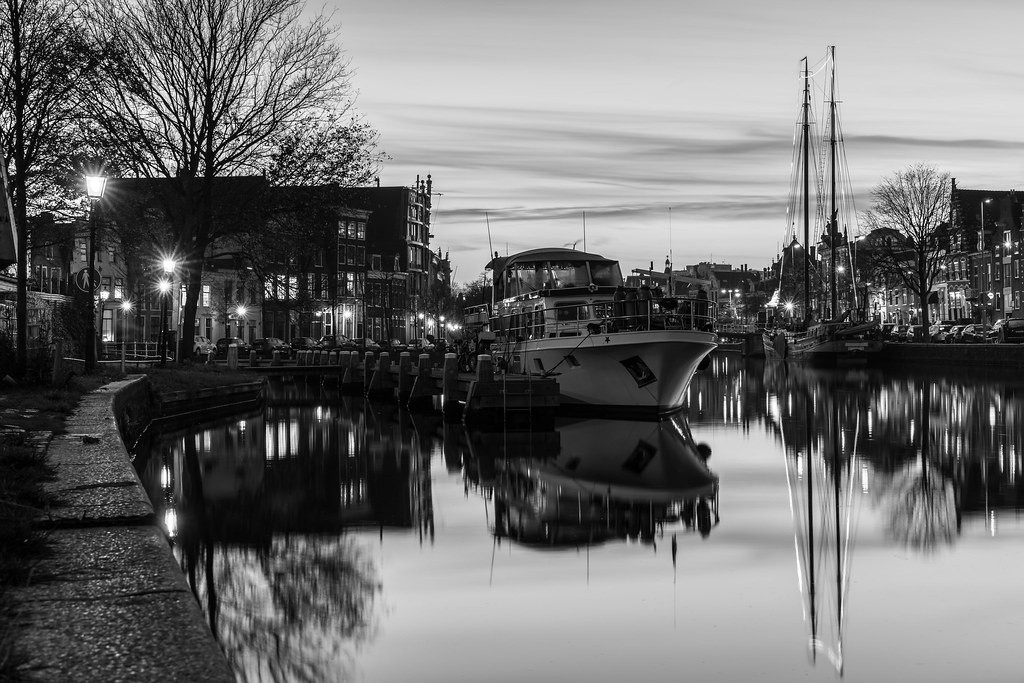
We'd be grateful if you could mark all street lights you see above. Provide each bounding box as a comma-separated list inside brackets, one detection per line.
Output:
[79, 160, 108, 377]
[980, 197, 993, 343]
[722, 286, 740, 308]
[87, 252, 466, 352]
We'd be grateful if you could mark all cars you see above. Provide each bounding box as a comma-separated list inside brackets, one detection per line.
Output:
[880, 319, 1024, 344]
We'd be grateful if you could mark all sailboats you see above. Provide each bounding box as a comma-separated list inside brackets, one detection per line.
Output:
[764, 354, 879, 676]
[757, 44, 878, 355]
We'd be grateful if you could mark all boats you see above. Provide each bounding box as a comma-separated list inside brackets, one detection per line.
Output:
[488, 408, 723, 550]
[453, 248, 722, 419]
